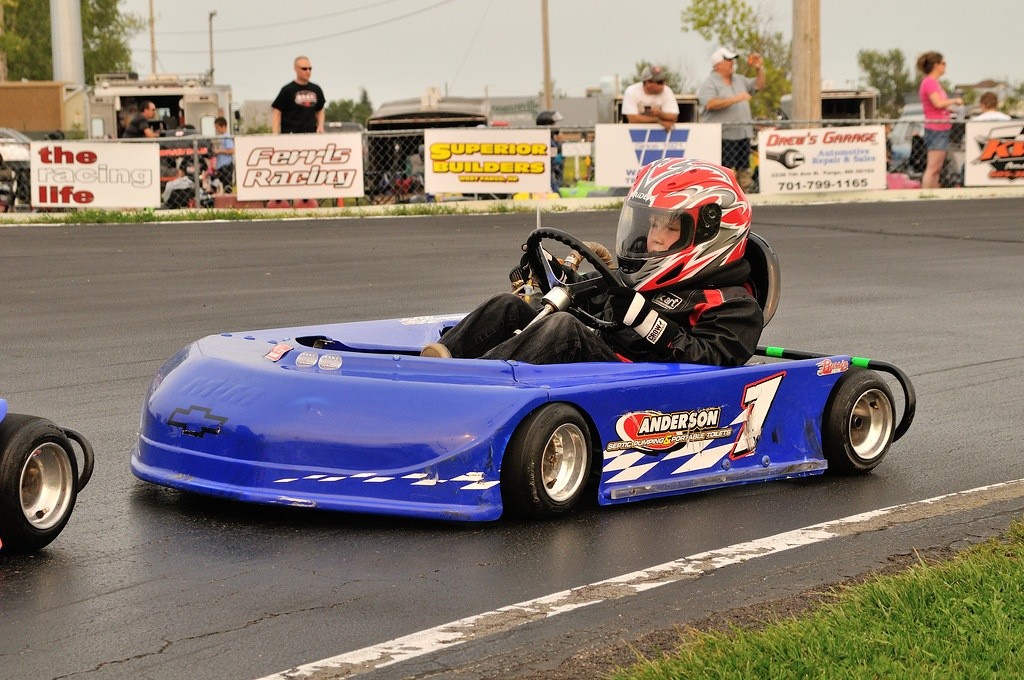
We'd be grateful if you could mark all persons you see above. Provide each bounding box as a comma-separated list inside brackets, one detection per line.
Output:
[419, 158, 763, 369]
[271, 57, 328, 133]
[621, 66, 679, 133]
[161, 161, 204, 203]
[951, 95, 966, 115]
[123, 102, 159, 138]
[698, 48, 766, 179]
[881, 123, 892, 170]
[973, 92, 1012, 120]
[212, 117, 234, 193]
[916, 52, 964, 188]
[536, 110, 563, 197]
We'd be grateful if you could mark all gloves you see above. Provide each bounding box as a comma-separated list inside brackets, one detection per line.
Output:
[520, 243, 574, 291]
[606, 286, 679, 354]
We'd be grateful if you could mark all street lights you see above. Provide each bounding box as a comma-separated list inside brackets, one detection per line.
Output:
[208, 9, 220, 85]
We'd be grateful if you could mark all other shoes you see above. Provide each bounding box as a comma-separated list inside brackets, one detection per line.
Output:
[420, 343, 452, 358]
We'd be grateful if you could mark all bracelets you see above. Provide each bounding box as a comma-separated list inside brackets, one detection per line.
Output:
[757, 65, 764, 70]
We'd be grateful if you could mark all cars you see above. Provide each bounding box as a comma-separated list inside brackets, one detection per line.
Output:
[0, 126, 39, 202]
[885, 101, 966, 176]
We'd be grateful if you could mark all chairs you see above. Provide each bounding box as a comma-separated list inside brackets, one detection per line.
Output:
[738, 231, 782, 327]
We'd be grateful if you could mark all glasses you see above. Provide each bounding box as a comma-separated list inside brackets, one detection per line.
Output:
[651, 80, 664, 85]
[300, 67, 311, 71]
[941, 62, 945, 66]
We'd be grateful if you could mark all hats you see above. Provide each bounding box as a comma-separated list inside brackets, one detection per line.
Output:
[641, 65, 672, 82]
[710, 48, 740, 66]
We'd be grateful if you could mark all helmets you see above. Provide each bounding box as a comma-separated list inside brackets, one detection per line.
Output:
[616, 157, 752, 300]
[536, 111, 563, 126]
